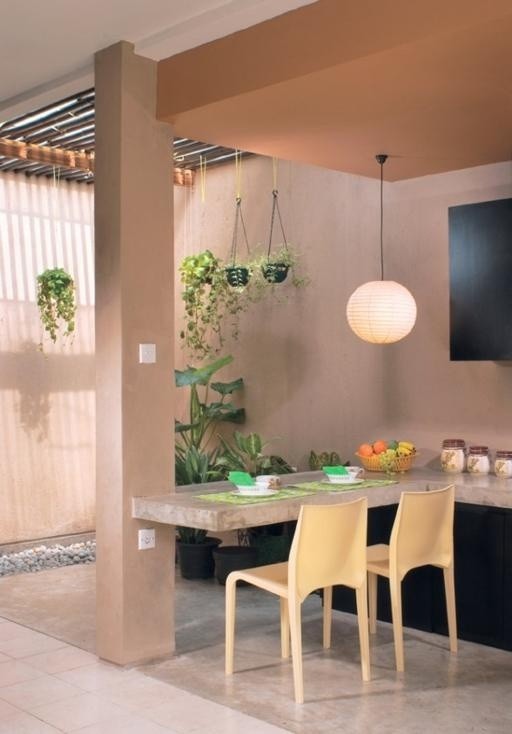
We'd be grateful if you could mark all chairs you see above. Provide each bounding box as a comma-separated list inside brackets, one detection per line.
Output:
[365, 484, 459, 672]
[223, 496, 371, 705]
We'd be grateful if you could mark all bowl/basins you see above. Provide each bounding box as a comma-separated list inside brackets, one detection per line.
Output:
[257, 475, 278, 488]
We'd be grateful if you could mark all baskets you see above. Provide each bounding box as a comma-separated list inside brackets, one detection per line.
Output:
[355, 452, 420, 472]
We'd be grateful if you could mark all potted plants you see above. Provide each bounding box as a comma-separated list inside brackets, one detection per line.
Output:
[172, 354, 297, 589]
[31, 269, 77, 348]
[178, 246, 312, 361]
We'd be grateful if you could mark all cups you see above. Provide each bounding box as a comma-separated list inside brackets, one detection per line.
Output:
[345, 466, 364, 477]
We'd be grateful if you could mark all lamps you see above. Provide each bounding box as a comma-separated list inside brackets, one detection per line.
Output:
[346, 155, 418, 346]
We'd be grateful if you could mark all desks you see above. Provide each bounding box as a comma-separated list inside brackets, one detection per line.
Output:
[129, 460, 512, 534]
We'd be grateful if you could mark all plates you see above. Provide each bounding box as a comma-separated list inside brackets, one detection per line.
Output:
[327, 473, 358, 480]
[237, 482, 270, 492]
[321, 478, 364, 485]
[233, 490, 278, 498]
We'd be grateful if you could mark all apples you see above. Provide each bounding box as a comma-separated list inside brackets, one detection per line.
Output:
[373, 441, 387, 455]
[359, 443, 373, 456]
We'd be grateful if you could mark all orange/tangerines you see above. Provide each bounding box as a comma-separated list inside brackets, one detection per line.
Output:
[388, 440, 398, 450]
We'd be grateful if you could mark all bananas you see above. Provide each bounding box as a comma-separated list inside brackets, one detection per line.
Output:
[397, 442, 417, 457]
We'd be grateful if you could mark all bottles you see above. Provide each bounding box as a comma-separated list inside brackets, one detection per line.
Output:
[467, 446, 490, 475]
[493, 451, 512, 479]
[440, 439, 466, 473]
